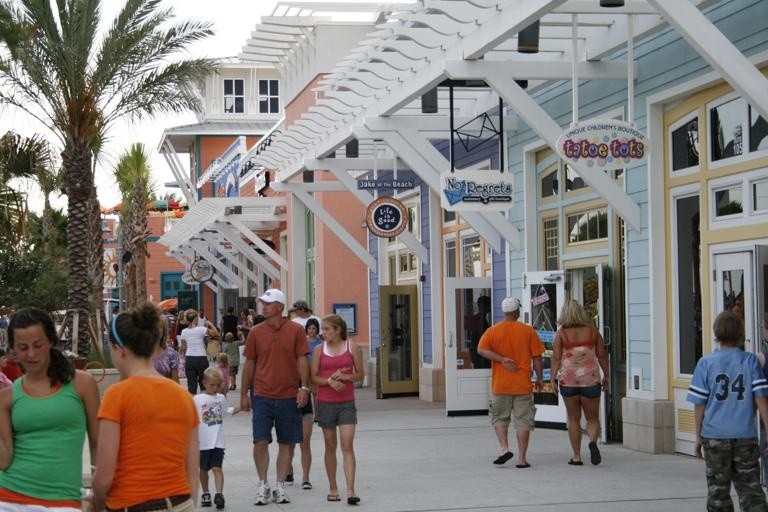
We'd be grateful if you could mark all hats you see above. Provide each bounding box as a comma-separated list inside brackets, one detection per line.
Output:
[287, 300, 309, 313]
[500, 297, 523, 313]
[257, 288, 287, 307]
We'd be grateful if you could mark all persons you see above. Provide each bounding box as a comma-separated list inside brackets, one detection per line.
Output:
[0, 307, 101, 512]
[290, 301, 360, 505]
[87, 302, 200, 512]
[0, 307, 24, 385]
[757, 315, 768, 487]
[239, 288, 312, 505]
[194, 368, 243, 509]
[153, 306, 266, 411]
[686, 310, 768, 512]
[466, 295, 492, 368]
[550, 299, 609, 465]
[476, 296, 546, 468]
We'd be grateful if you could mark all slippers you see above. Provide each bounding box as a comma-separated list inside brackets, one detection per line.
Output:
[347, 497, 360, 505]
[492, 451, 514, 464]
[326, 493, 341, 502]
[567, 458, 584, 466]
[587, 441, 602, 466]
[515, 461, 531, 468]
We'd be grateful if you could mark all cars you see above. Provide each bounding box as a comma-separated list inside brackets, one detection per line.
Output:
[52, 298, 125, 341]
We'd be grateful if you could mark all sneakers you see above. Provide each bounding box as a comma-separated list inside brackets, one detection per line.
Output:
[213, 492, 226, 509]
[301, 481, 313, 490]
[200, 492, 213, 507]
[285, 474, 295, 486]
[253, 478, 272, 507]
[270, 481, 292, 504]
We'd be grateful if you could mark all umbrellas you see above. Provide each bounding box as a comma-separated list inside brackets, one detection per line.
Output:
[97, 198, 188, 216]
